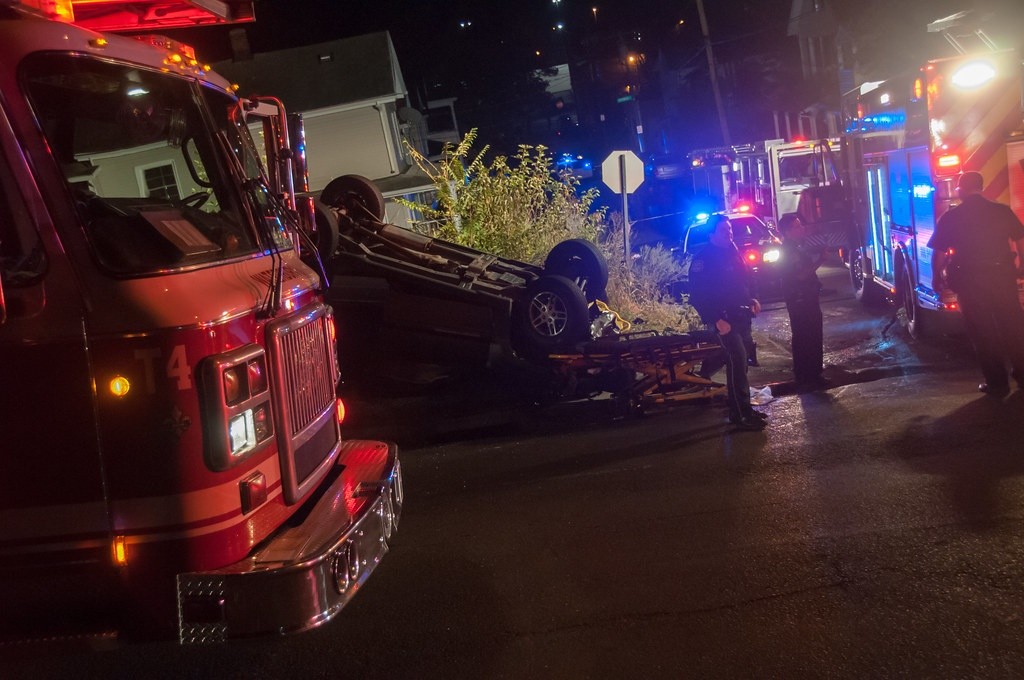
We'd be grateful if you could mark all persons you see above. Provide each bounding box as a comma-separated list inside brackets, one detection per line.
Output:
[774, 211, 829, 395]
[685, 214, 768, 431]
[926, 171, 1024, 397]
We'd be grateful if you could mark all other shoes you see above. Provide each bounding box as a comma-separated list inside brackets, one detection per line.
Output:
[728, 413, 768, 430]
[978, 383, 1010, 394]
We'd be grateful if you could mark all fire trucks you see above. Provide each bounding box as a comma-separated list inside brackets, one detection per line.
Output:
[0, 1, 403, 679]
[689, 138, 852, 264]
[808, 4, 1023, 340]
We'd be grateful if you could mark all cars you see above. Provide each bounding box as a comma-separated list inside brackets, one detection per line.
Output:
[672, 204, 783, 303]
[644, 150, 689, 185]
[551, 150, 594, 182]
[300, 176, 631, 381]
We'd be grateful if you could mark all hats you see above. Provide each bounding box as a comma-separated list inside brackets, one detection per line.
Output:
[953, 172, 983, 191]
